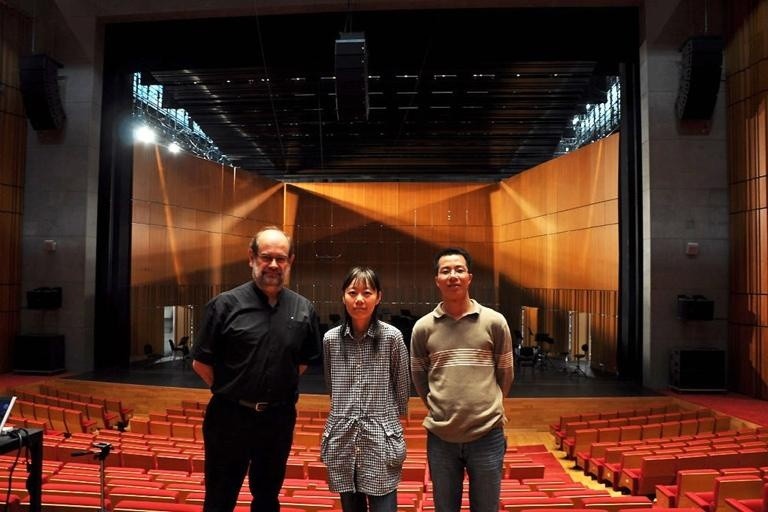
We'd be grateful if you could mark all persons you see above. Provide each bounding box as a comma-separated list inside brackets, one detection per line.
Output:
[410, 246, 516, 511]
[319, 265, 409, 512]
[186, 223, 324, 511]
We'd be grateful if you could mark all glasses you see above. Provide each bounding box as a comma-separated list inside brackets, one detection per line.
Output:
[252, 251, 290, 264]
[432, 268, 471, 279]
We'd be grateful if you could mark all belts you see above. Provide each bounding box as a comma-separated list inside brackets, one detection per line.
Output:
[216, 390, 299, 413]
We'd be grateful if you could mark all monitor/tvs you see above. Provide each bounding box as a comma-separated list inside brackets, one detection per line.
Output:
[0, 396, 17, 434]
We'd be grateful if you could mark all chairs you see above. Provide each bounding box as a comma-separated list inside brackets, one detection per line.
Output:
[574, 344, 589, 377]
[520, 351, 539, 377]
[169, 336, 191, 370]
[0, 383, 768, 512]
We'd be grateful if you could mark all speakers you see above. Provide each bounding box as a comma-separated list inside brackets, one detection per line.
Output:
[27, 289, 64, 312]
[18, 54, 67, 133]
[677, 296, 714, 321]
[334, 39, 371, 123]
[669, 348, 727, 388]
[14, 332, 66, 371]
[675, 35, 726, 120]
